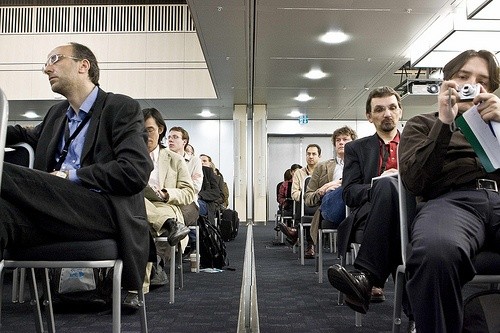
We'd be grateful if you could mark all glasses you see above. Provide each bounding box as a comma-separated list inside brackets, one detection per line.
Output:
[42, 54, 83, 75]
[147, 127, 160, 135]
[167, 136, 183, 140]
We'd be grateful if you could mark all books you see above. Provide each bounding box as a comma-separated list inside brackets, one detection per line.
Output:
[371, 172, 399, 189]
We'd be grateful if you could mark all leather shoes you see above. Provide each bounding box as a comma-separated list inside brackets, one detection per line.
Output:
[182, 241, 197, 263]
[304, 241, 315, 258]
[327, 263, 375, 315]
[278, 222, 298, 246]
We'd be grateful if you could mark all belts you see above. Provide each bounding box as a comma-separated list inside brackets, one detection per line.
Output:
[457, 178, 500, 192]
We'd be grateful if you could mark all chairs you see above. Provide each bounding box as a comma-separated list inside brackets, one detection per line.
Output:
[0, 90, 201, 333]
[274, 143, 500, 333]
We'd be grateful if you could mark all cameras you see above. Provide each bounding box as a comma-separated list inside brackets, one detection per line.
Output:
[457, 84, 481, 103]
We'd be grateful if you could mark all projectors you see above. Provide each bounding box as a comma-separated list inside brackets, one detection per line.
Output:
[403, 82, 441, 95]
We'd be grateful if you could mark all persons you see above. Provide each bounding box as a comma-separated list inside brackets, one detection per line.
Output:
[122, 108, 195, 309]
[327, 87, 417, 333]
[399, 49, 500, 333]
[0, 42, 159, 288]
[185, 144, 229, 217]
[280, 144, 321, 258]
[305, 127, 386, 302]
[149, 127, 203, 285]
[274, 164, 303, 244]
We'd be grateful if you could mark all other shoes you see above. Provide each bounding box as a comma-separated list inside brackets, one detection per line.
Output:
[274, 225, 281, 231]
[371, 286, 386, 300]
[164, 219, 191, 246]
[121, 291, 139, 310]
[149, 270, 169, 285]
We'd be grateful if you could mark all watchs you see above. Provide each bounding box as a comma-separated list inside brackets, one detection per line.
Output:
[55, 170, 69, 179]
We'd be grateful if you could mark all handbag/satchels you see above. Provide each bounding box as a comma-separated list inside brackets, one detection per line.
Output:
[42, 267, 112, 312]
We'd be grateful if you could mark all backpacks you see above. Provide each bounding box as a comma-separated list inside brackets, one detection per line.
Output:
[220, 208, 239, 241]
[197, 214, 229, 270]
